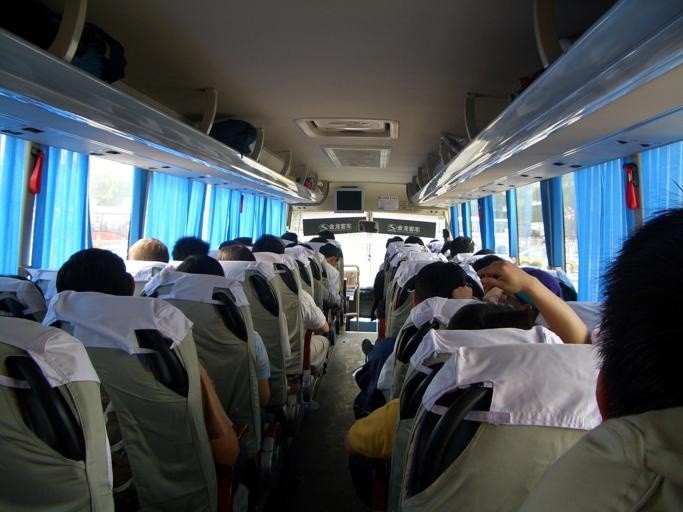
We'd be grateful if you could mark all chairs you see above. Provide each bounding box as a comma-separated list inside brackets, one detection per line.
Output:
[378, 241, 682, 511]
[0, 240, 344, 512]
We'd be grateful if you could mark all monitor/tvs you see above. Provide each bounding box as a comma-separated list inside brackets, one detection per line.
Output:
[334, 188, 365, 213]
